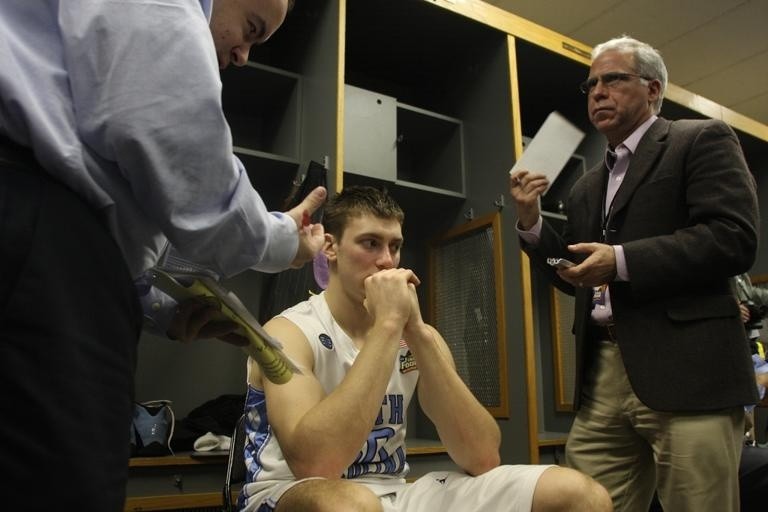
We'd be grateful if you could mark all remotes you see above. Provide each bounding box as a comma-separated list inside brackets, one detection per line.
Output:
[546, 257, 576, 270]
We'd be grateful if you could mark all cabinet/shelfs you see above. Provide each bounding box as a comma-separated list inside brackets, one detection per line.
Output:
[231, 60, 303, 165]
[343, 82, 467, 208]
[522, 135, 586, 221]
[122, 0, 768, 511]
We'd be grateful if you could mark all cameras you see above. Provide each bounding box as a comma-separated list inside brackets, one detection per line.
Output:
[740, 298, 768, 329]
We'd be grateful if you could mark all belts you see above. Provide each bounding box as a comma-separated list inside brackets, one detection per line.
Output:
[588, 321, 618, 344]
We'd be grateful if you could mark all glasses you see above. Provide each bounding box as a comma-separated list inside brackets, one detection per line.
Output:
[578, 72, 652, 95]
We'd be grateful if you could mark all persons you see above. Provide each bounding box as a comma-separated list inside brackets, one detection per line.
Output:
[508, 32, 763, 512]
[234, 183, 616, 511]
[0, 0, 331, 512]
[734, 269, 767, 447]
